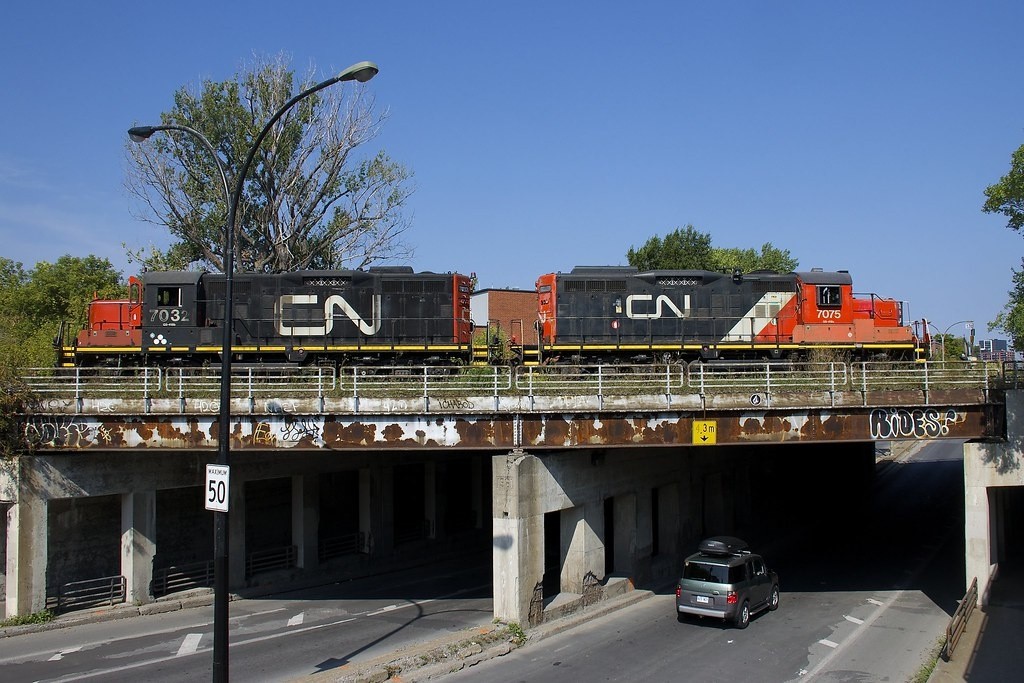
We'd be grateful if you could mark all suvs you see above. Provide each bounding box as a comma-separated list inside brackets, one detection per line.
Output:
[676, 536, 780, 629]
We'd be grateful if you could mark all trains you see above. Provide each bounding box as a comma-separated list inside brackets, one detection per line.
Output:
[56, 266, 474, 375]
[533, 264, 918, 374]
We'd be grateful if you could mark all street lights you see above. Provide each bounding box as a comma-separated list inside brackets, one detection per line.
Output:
[928, 318, 975, 367]
[123, 59, 380, 683]
